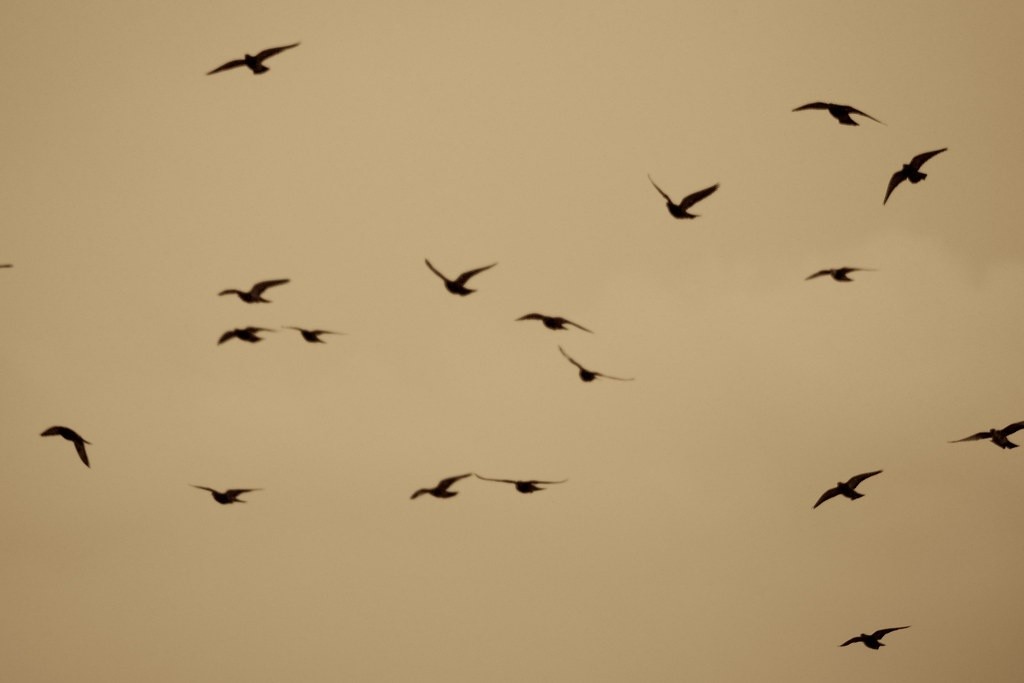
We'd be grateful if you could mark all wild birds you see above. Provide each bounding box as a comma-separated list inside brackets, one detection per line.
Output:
[409, 472, 472, 500]
[425, 258, 499, 297]
[814, 470, 883, 510]
[190, 484, 264, 505]
[217, 279, 290, 304]
[206, 42, 302, 77]
[557, 345, 635, 383]
[514, 313, 595, 334]
[40, 427, 92, 470]
[839, 626, 911, 650]
[474, 473, 569, 494]
[947, 421, 1024, 450]
[646, 174, 721, 221]
[217, 327, 276, 347]
[882, 147, 948, 207]
[282, 325, 341, 344]
[801, 267, 881, 283]
[792, 102, 889, 128]
